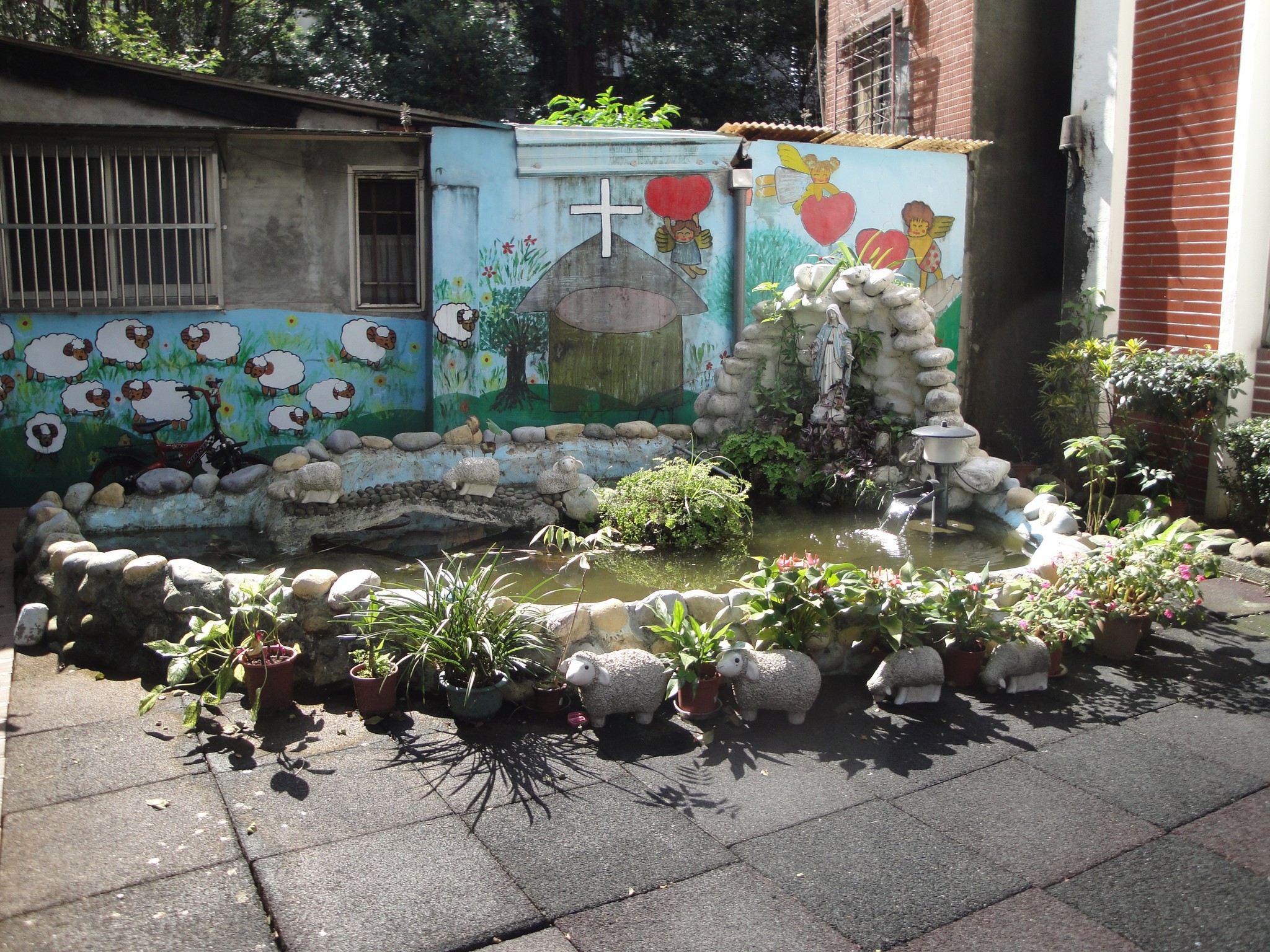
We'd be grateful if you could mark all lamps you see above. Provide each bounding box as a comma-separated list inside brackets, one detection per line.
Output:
[911, 421, 976, 527]
[1058, 114, 1084, 150]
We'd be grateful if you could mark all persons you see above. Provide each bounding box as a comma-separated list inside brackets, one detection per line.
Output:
[802, 303, 855, 412]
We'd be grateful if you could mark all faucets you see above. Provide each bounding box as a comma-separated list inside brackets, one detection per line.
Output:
[893, 478, 942, 505]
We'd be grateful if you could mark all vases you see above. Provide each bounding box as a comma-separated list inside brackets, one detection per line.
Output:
[943, 611, 1150, 684]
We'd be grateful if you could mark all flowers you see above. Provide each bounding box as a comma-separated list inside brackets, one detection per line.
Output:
[725, 517, 1206, 646]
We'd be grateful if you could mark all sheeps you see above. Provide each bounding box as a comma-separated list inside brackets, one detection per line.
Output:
[438, 456, 500, 499]
[977, 634, 1052, 695]
[534, 454, 584, 495]
[283, 459, 343, 505]
[864, 645, 945, 706]
[713, 647, 824, 726]
[557, 646, 670, 729]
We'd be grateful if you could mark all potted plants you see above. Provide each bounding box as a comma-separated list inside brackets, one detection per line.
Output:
[326, 542, 736, 720]
[995, 422, 1040, 488]
[140, 567, 302, 727]
